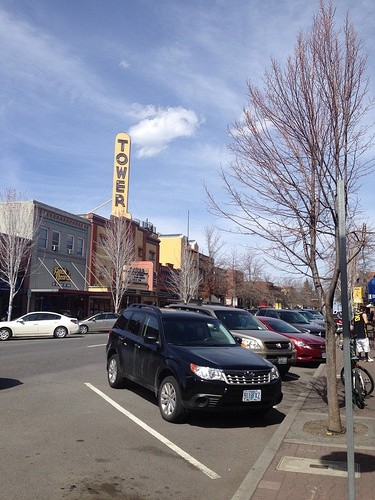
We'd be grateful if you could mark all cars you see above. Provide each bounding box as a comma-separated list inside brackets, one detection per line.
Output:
[78, 312, 120, 334]
[0, 312, 80, 341]
[254, 315, 326, 366]
[299, 308, 344, 336]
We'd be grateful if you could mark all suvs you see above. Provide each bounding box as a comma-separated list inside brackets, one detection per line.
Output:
[106, 303, 283, 424]
[254, 307, 326, 339]
[165, 302, 297, 378]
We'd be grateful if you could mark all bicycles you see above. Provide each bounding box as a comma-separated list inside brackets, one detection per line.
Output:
[341, 334, 375, 409]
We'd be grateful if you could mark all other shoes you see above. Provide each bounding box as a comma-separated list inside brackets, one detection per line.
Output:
[365, 358, 373, 362]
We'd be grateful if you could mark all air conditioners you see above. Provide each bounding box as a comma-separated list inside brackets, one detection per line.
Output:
[67, 249, 73, 255]
[52, 245, 60, 251]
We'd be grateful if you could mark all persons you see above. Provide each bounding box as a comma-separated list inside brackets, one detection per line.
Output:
[354, 307, 374, 361]
